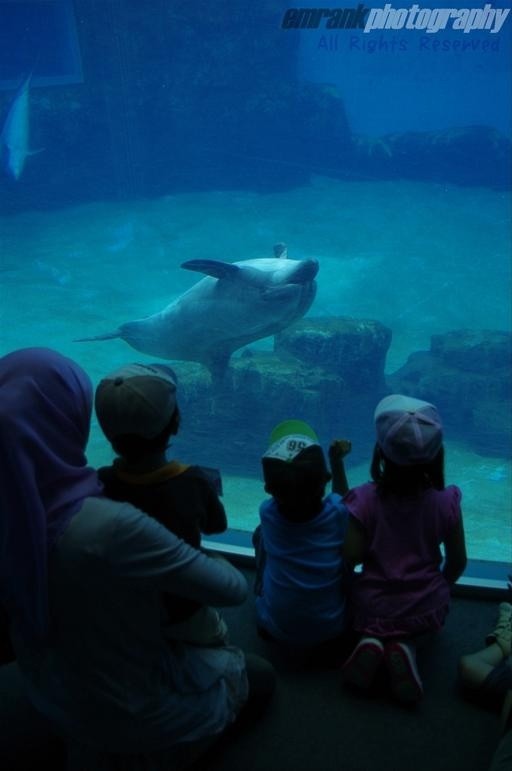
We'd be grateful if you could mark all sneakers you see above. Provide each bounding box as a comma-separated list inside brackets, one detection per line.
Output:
[335, 639, 384, 697]
[388, 645, 422, 710]
[485, 604, 511, 656]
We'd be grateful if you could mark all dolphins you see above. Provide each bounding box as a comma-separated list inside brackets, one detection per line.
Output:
[71, 241, 321, 384]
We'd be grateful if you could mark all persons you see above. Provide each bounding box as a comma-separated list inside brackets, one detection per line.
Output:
[94, 362, 231, 652]
[326, 396, 465, 705]
[252, 421, 350, 658]
[0, 347, 246, 769]
[461, 601, 512, 771]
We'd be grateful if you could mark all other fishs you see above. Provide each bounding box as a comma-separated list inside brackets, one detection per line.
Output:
[2, 70, 50, 182]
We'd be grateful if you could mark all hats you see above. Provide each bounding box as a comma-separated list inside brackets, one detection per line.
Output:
[374, 393, 442, 465]
[261, 418, 327, 501]
[96, 362, 177, 444]
